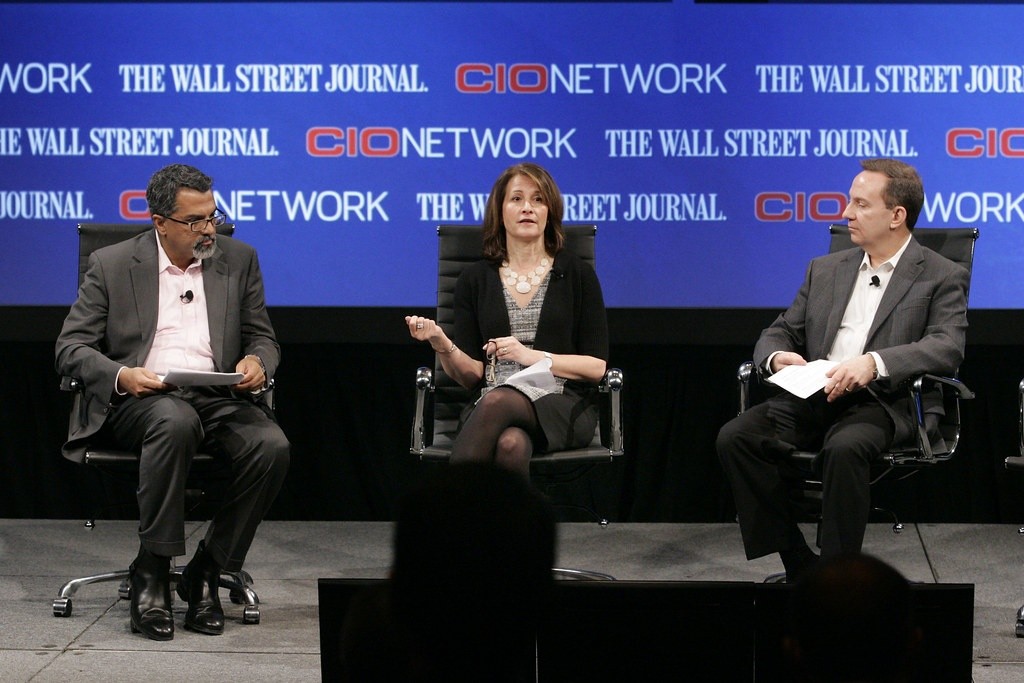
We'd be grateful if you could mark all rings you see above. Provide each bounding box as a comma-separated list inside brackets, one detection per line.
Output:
[503, 347, 507, 354]
[845, 385, 853, 392]
[416, 324, 423, 329]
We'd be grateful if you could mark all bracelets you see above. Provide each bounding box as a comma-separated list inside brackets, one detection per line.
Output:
[431, 344, 454, 354]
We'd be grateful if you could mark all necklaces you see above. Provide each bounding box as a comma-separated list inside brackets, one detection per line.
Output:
[500, 257, 549, 294]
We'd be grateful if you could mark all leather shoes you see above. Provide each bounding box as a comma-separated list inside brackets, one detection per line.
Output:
[176, 540, 224, 635]
[129, 543, 175, 640]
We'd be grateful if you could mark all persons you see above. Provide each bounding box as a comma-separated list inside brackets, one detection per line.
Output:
[716, 158, 972, 582]
[405, 163, 612, 471]
[55, 166, 291, 641]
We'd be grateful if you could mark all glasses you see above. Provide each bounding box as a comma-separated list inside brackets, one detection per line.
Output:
[162, 207, 227, 231]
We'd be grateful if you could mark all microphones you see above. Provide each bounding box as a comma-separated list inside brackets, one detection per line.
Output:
[186, 291, 193, 301]
[555, 266, 564, 277]
[872, 275, 881, 287]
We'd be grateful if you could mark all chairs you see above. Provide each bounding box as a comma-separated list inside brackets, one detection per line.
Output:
[737, 221, 979, 580]
[409, 225, 625, 580]
[52, 222, 274, 624]
